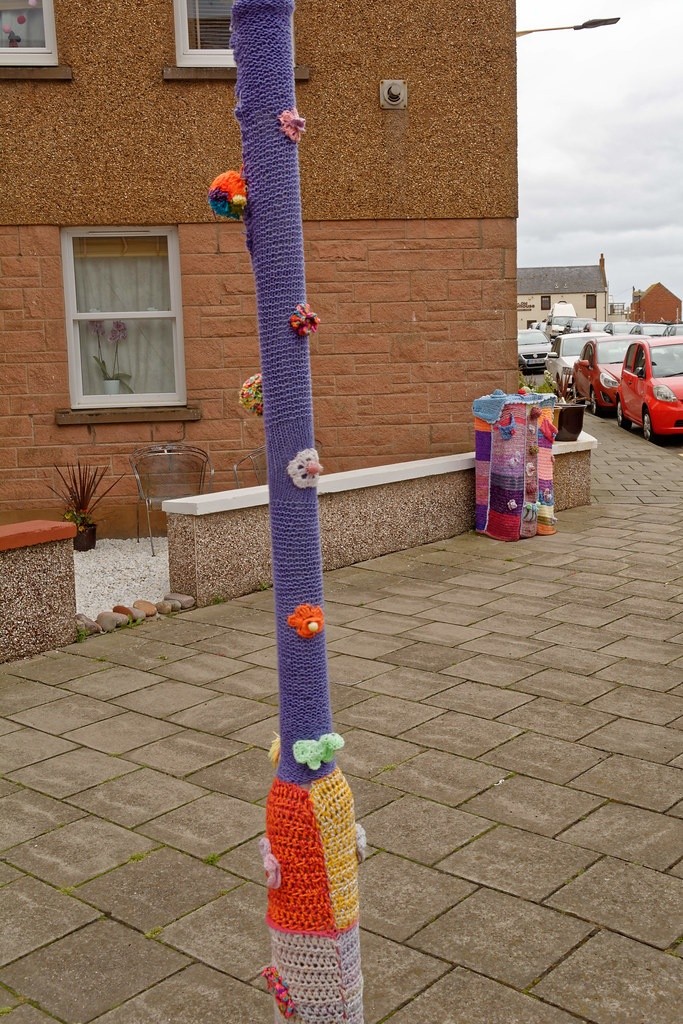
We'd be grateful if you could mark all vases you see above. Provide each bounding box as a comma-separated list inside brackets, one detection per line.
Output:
[103, 379, 120, 395]
[74, 524, 96, 551]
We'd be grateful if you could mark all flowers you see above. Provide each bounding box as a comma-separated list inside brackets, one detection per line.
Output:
[87, 310, 133, 393]
[44, 460, 127, 533]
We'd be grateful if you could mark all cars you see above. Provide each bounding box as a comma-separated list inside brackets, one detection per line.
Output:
[518, 329, 554, 373]
[571, 336, 654, 415]
[616, 336, 683, 442]
[529, 317, 683, 358]
[545, 332, 615, 387]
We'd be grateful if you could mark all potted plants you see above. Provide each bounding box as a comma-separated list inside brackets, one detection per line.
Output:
[554, 368, 587, 442]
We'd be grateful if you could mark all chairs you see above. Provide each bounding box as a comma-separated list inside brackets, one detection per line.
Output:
[127, 443, 212, 558]
[233, 443, 269, 489]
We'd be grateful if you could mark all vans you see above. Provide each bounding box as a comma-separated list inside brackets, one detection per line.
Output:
[547, 303, 576, 343]
